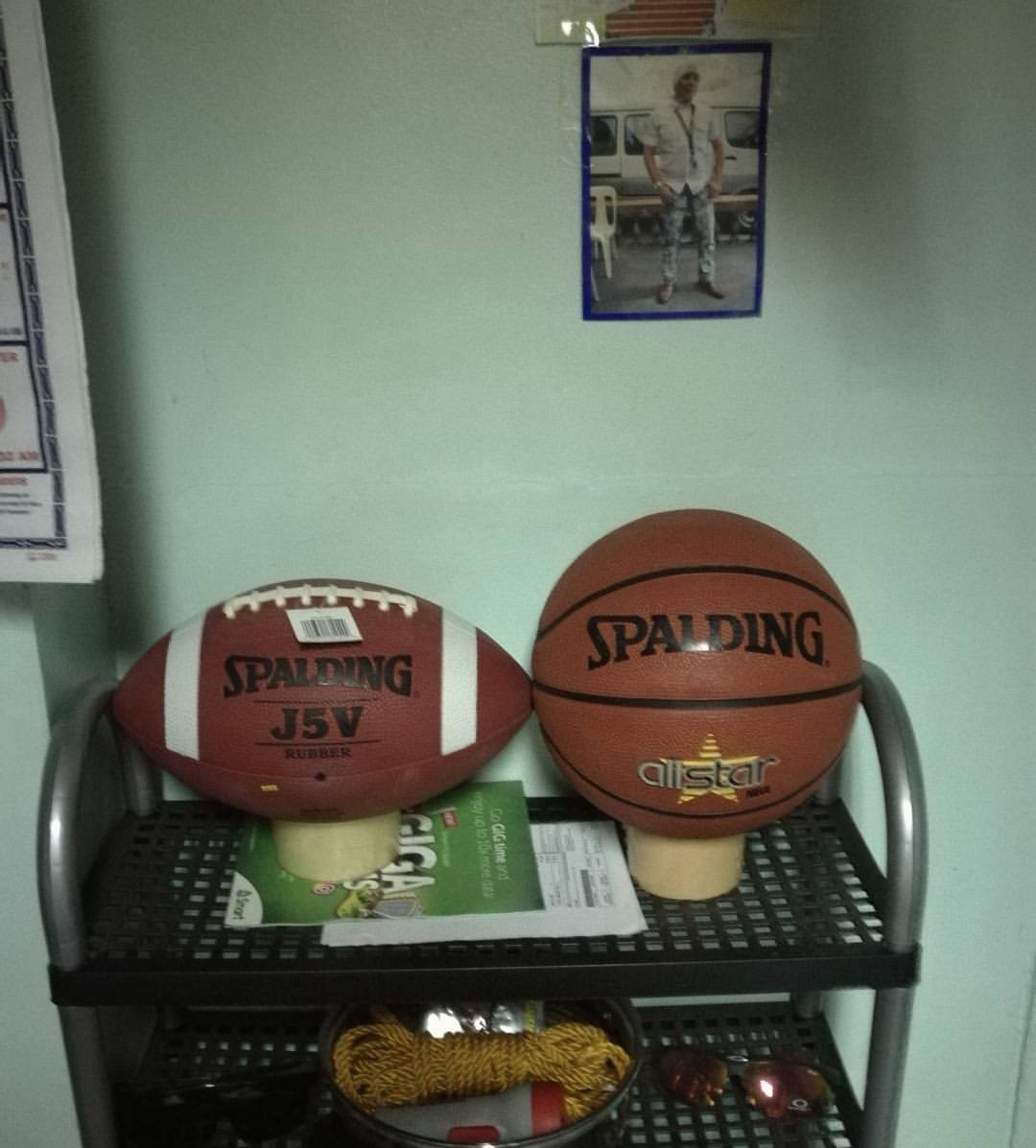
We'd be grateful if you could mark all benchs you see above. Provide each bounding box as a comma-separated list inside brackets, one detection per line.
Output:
[616, 194, 758, 242]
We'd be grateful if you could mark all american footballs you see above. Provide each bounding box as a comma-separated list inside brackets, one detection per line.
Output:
[112, 578, 533, 825]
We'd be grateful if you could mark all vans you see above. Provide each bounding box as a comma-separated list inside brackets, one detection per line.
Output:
[590, 105, 759, 234]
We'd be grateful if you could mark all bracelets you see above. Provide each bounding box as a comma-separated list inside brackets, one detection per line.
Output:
[651, 181, 665, 190]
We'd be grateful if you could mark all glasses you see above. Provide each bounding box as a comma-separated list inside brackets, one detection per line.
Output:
[654, 1047, 839, 1124]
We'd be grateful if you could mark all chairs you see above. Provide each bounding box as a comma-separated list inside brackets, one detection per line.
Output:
[590, 185, 618, 278]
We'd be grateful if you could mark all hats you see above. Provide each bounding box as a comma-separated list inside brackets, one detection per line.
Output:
[670, 61, 700, 87]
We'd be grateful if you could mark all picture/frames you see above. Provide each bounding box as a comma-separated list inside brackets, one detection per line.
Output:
[582, 42, 772, 320]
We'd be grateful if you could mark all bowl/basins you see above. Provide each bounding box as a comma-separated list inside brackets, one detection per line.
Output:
[321, 994, 642, 1148]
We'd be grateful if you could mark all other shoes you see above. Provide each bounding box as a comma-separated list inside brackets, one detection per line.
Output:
[699, 279, 725, 299]
[657, 281, 675, 302]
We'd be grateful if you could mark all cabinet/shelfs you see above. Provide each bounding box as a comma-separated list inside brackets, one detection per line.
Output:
[39, 662, 929, 1147]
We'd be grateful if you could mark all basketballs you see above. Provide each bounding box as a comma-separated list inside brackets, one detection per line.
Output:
[530, 508, 864, 840]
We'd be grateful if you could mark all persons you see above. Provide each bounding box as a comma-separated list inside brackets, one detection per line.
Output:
[636, 60, 725, 304]
